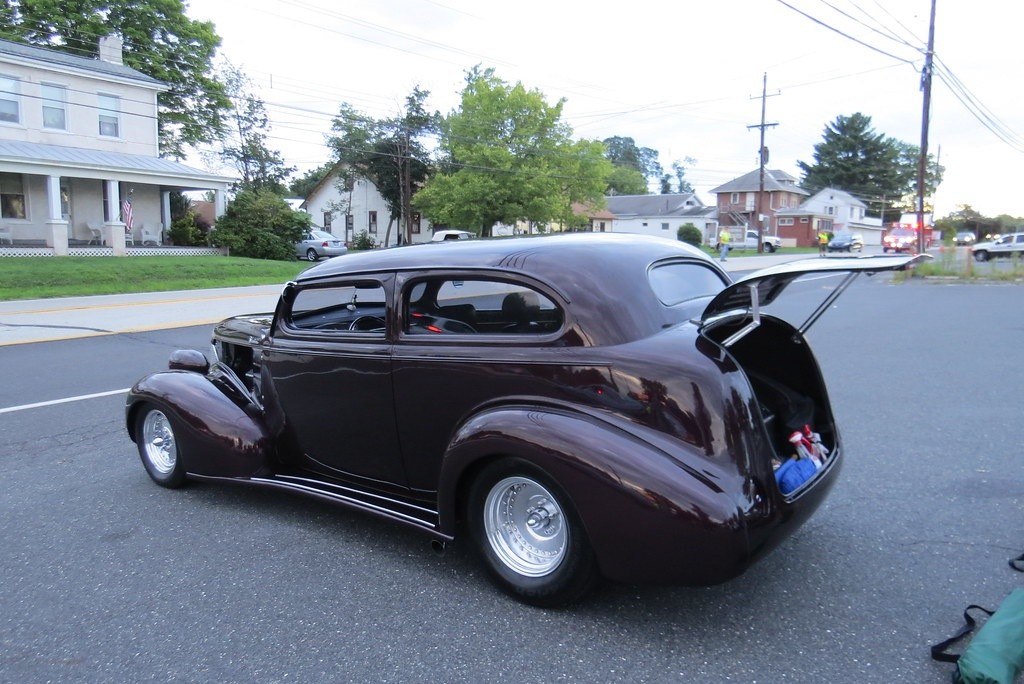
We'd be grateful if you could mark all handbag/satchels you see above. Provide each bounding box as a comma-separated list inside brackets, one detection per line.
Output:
[931, 587, 1024, 684]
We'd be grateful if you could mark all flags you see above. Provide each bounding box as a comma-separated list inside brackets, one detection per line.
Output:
[122, 191, 135, 230]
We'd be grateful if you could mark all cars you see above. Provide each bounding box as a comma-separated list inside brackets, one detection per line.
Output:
[295, 231, 347, 262]
[126, 230, 934, 609]
[827, 233, 865, 253]
[952, 232, 976, 246]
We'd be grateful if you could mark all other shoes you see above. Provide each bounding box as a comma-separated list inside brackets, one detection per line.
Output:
[721, 260, 727, 262]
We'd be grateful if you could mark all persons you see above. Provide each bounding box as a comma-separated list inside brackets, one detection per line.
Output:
[720, 226, 731, 261]
[817, 229, 829, 257]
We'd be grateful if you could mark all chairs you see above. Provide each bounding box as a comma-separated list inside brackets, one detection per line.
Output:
[501, 291, 540, 333]
[86, 222, 134, 246]
[140, 222, 163, 246]
[0, 220, 15, 245]
[439, 304, 476, 335]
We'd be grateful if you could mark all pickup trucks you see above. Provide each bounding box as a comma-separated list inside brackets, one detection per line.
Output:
[709, 230, 782, 253]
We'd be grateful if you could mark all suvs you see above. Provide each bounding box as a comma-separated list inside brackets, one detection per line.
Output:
[970, 232, 1024, 262]
[883, 223, 930, 253]
